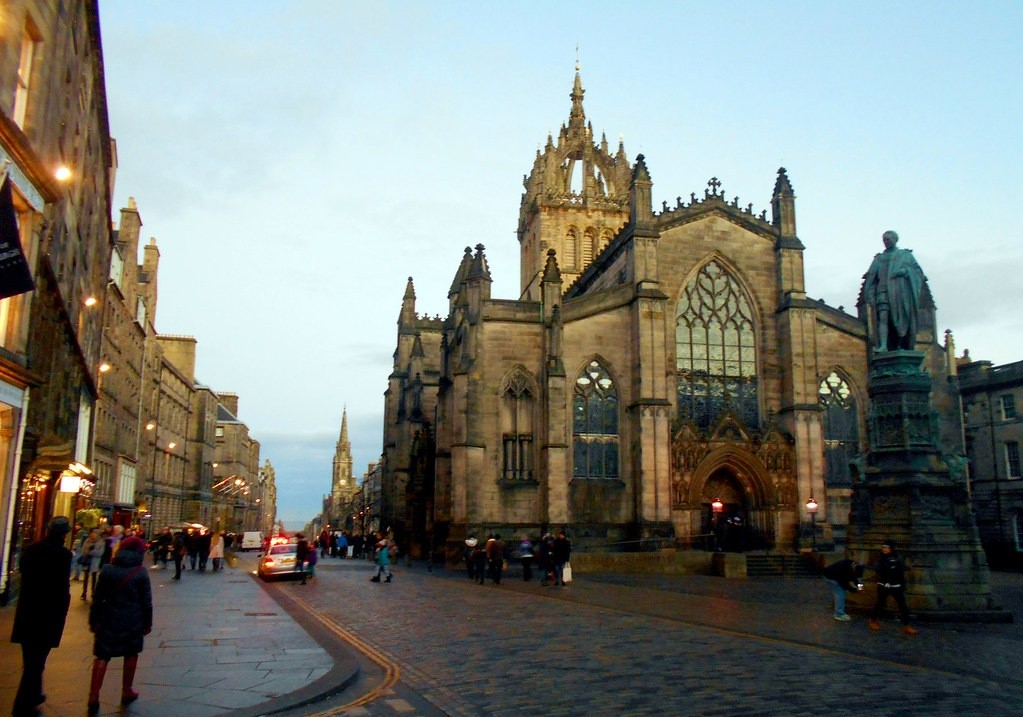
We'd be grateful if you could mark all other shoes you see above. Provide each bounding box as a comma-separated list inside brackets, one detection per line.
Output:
[35, 695, 46, 706]
[12, 707, 43, 717]
[81, 593, 86, 599]
[73, 575, 79, 581]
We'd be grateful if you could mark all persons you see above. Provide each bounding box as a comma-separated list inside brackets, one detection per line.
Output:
[869, 543, 916, 634]
[855, 230, 936, 353]
[72, 524, 149, 600]
[519, 537, 535, 581]
[11, 516, 73, 717]
[824, 559, 865, 621]
[319, 530, 377, 560]
[294, 535, 318, 585]
[148, 526, 224, 579]
[88, 537, 153, 717]
[463, 534, 506, 584]
[223, 533, 245, 552]
[537, 533, 571, 586]
[371, 533, 393, 583]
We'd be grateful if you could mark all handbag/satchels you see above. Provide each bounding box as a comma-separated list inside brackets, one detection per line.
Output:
[562, 561, 572, 582]
[77, 554, 91, 567]
[502, 560, 507, 571]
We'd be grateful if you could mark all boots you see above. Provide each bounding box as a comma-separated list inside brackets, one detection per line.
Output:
[370, 576, 380, 582]
[384, 576, 391, 583]
[87, 658, 108, 712]
[121, 655, 139, 702]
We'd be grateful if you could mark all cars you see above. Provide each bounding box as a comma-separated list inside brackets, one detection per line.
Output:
[241, 532, 265, 552]
[257, 537, 314, 579]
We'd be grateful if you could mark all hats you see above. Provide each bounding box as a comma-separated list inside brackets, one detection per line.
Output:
[379, 539, 386, 546]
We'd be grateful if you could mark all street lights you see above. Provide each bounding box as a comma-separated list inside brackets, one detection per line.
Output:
[807, 490, 818, 548]
[216, 516, 220, 533]
